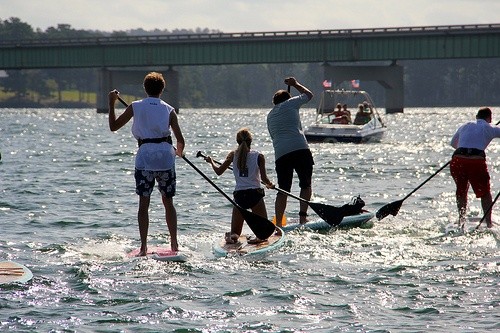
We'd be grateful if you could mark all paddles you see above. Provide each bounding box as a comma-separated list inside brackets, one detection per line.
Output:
[285, 81, 293, 97]
[374, 120, 500, 221]
[195, 150, 345, 228]
[114, 89, 277, 242]
[476, 192, 500, 229]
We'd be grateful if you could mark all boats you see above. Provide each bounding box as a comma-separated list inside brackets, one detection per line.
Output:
[127, 245, 191, 263]
[445, 216, 500, 238]
[303, 89, 389, 141]
[277, 208, 376, 233]
[212, 224, 286, 258]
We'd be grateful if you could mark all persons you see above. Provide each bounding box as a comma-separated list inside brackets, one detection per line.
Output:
[450, 107, 500, 228]
[267, 77, 315, 234]
[108, 72, 185, 257]
[328, 100, 373, 125]
[205, 128, 276, 244]
